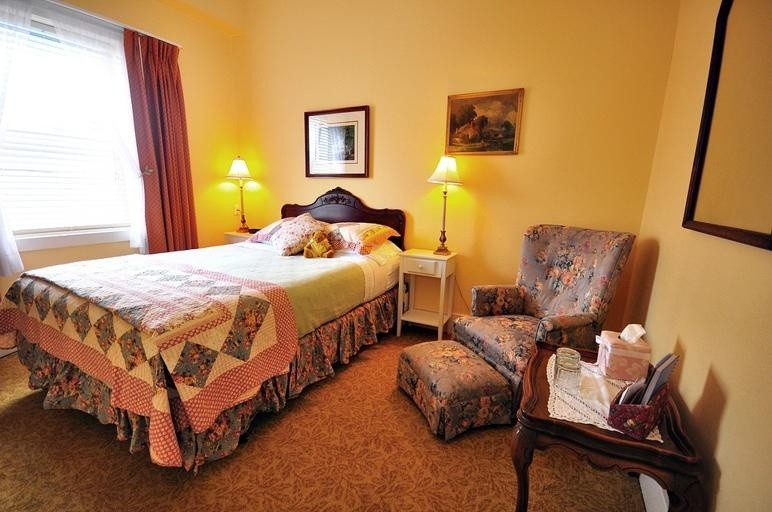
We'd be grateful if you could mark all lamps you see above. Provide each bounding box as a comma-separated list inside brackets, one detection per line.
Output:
[227, 156, 253, 234]
[428, 156, 463, 257]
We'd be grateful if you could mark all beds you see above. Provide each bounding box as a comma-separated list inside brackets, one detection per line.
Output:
[5, 186, 410, 473]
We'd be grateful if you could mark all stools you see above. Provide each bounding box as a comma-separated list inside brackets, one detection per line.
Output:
[396, 341, 514, 441]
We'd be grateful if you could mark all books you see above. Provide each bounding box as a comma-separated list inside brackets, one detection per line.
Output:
[641, 353, 679, 404]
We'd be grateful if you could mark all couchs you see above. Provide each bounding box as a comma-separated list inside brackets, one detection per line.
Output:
[455, 225, 637, 423]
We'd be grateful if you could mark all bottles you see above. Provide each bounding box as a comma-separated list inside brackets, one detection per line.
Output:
[553, 348, 582, 390]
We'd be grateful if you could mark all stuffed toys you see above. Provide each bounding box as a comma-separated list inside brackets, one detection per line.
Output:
[303, 231, 334, 258]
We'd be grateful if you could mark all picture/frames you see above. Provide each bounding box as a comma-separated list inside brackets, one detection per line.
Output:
[444, 88, 525, 155]
[304, 105, 370, 179]
[681, 0, 772, 249]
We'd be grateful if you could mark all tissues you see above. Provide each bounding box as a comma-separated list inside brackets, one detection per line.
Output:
[596, 323, 651, 385]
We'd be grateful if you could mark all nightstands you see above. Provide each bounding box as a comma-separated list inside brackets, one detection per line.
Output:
[225, 230, 249, 244]
[398, 248, 455, 340]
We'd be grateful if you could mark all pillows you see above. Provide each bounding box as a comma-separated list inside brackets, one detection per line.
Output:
[245, 216, 337, 243]
[270, 213, 325, 256]
[325, 222, 401, 255]
